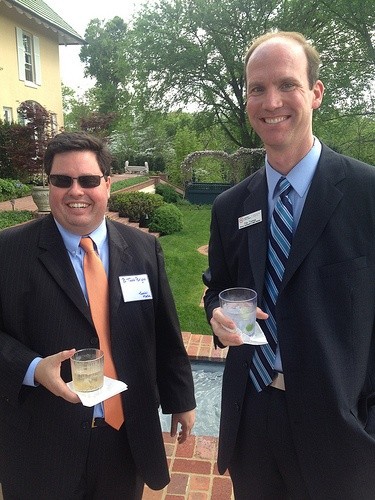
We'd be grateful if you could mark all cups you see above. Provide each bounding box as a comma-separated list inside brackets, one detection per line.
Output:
[70, 349, 105, 392]
[220, 287, 257, 336]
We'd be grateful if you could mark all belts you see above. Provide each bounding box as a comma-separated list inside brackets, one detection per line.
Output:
[92, 417, 110, 428]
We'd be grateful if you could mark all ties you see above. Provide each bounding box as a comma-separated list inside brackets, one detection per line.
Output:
[79, 238, 124, 431]
[250, 176, 293, 393]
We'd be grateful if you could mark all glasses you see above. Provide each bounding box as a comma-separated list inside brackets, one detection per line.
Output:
[48, 174, 105, 188]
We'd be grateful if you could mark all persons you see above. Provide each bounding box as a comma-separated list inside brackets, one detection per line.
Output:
[0, 130, 196, 500]
[200, 31, 375, 500]
